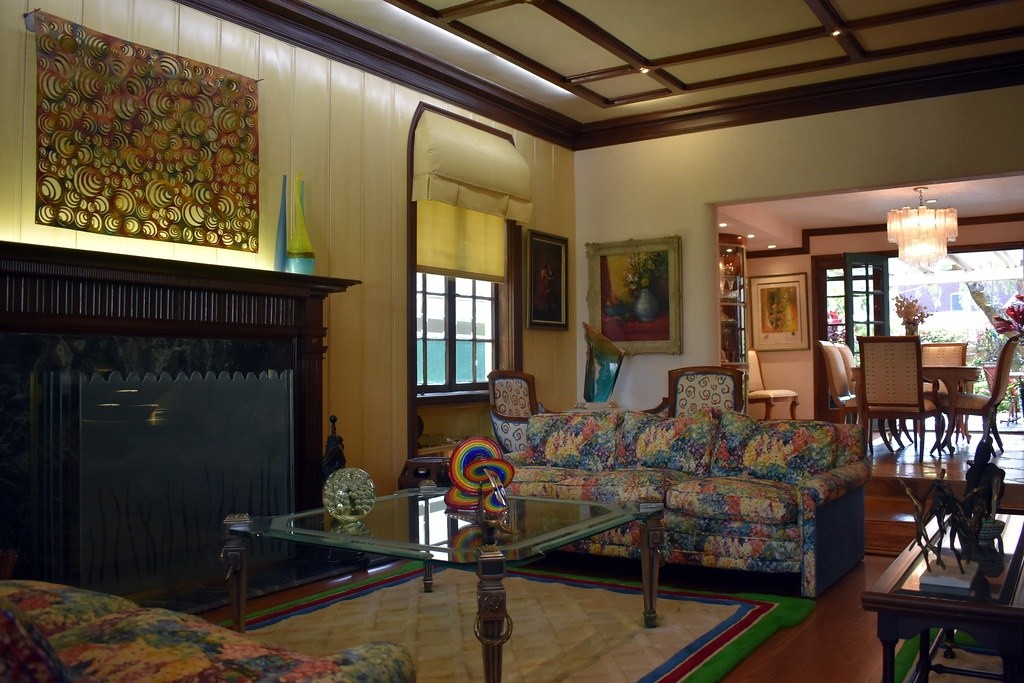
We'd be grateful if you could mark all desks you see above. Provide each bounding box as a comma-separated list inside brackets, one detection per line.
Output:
[1009, 370, 1023, 382]
[860, 507, 1024, 683]
[849, 365, 982, 455]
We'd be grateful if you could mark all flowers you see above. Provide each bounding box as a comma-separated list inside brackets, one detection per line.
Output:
[892, 294, 934, 328]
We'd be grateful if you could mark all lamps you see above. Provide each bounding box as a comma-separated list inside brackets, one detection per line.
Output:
[886, 186, 959, 269]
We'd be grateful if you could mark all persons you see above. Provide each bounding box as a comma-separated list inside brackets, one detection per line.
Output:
[920, 468, 957, 567]
[893, 478, 944, 568]
[950, 441, 1001, 574]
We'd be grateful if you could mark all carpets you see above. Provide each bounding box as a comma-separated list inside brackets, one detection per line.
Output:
[220, 555, 816, 683]
[881, 628, 1003, 683]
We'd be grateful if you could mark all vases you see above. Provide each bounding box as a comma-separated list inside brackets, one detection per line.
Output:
[284, 172, 315, 275]
[904, 324, 919, 336]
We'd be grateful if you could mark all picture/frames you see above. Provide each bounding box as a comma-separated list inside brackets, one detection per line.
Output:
[748, 271, 811, 353]
[527, 228, 570, 329]
[584, 234, 682, 357]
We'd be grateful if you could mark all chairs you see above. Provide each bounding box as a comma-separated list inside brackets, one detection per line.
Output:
[485, 370, 567, 454]
[641, 367, 748, 418]
[747, 349, 799, 420]
[815, 337, 1019, 463]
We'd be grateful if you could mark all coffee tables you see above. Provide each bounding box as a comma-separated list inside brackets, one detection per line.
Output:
[222, 480, 666, 683]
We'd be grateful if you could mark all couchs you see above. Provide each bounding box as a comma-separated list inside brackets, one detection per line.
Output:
[0, 579, 418, 683]
[398, 411, 873, 598]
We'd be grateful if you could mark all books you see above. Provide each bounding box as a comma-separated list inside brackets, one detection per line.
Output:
[919, 554, 978, 589]
